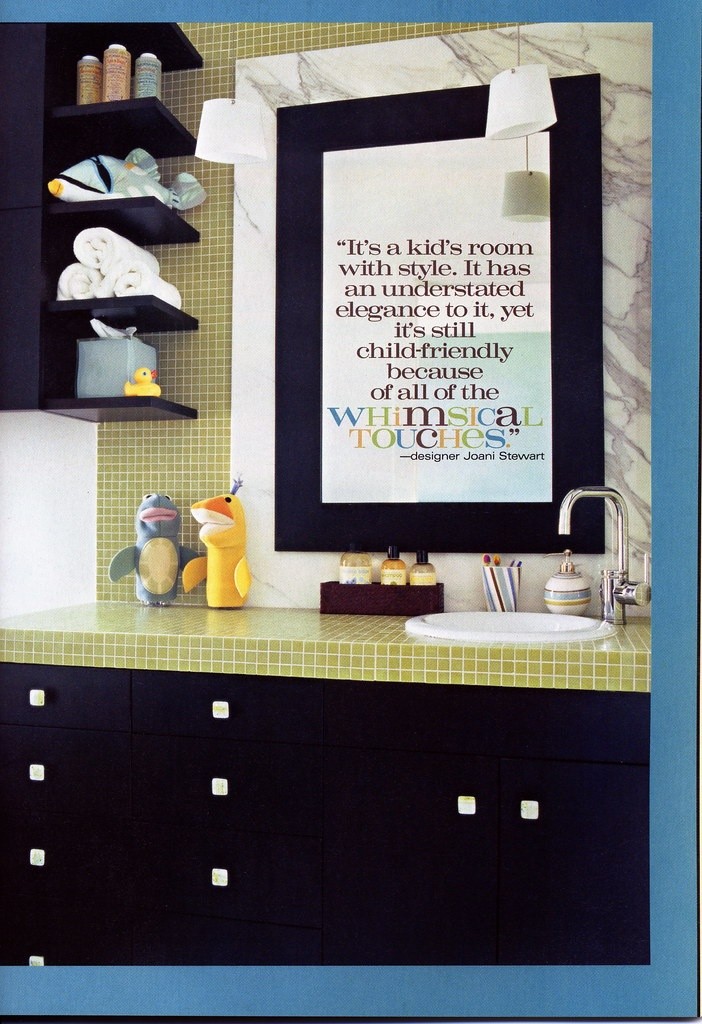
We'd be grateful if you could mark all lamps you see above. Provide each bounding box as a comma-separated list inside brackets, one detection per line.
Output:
[193, 96, 268, 166]
[499, 24, 550, 223]
[485, 62, 557, 141]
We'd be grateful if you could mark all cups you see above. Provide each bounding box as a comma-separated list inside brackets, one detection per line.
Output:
[482, 567, 520, 612]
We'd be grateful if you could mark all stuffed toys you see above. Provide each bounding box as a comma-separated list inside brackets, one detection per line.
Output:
[182, 479, 251, 607]
[109, 494, 200, 606]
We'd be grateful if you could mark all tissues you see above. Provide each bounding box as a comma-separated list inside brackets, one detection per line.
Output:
[74, 316, 158, 400]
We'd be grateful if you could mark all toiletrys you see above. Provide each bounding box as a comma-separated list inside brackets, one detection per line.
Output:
[338, 540, 373, 586]
[408, 547, 437, 586]
[541, 547, 592, 617]
[132, 52, 163, 105]
[380, 544, 407, 586]
[76, 43, 132, 106]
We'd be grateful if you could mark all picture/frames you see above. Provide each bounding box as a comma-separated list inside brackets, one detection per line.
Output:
[268, 72, 609, 556]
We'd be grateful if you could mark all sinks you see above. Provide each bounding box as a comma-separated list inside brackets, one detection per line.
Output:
[403, 610, 618, 644]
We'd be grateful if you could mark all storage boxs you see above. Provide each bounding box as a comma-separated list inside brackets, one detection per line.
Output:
[319, 582, 444, 616]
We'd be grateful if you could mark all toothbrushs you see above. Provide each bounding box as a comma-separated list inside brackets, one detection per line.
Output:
[481, 553, 502, 568]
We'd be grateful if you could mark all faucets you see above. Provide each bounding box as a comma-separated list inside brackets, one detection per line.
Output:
[557, 485, 651, 626]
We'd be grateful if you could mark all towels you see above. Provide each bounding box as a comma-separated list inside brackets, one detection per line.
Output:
[54, 225, 183, 312]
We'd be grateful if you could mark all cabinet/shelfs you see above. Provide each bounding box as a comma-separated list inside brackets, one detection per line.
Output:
[0, 22, 205, 424]
[322, 678, 650, 965]
[0, 663, 326, 966]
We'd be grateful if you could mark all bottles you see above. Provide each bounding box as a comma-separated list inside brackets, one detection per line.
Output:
[339, 541, 373, 584]
[102, 44, 131, 103]
[410, 549, 437, 585]
[381, 546, 407, 585]
[134, 53, 162, 102]
[76, 56, 103, 105]
